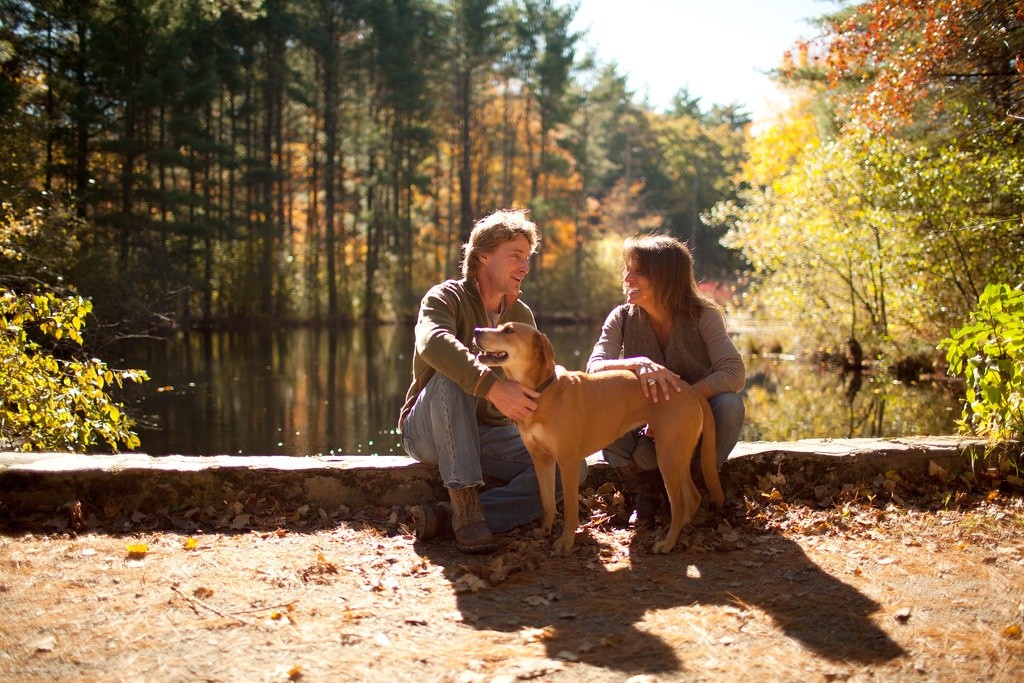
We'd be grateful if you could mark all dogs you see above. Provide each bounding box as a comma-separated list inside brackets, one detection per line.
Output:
[472, 321, 724, 557]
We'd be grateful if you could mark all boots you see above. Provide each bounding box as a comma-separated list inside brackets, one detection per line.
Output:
[615, 464, 653, 526]
[719, 462, 746, 517]
[417, 504, 447, 540]
[448, 485, 499, 552]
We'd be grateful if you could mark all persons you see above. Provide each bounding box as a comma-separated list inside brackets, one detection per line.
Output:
[396, 207, 588, 550]
[584, 231, 748, 529]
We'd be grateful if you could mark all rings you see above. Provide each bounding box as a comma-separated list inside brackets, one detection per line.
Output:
[647, 379, 656, 385]
